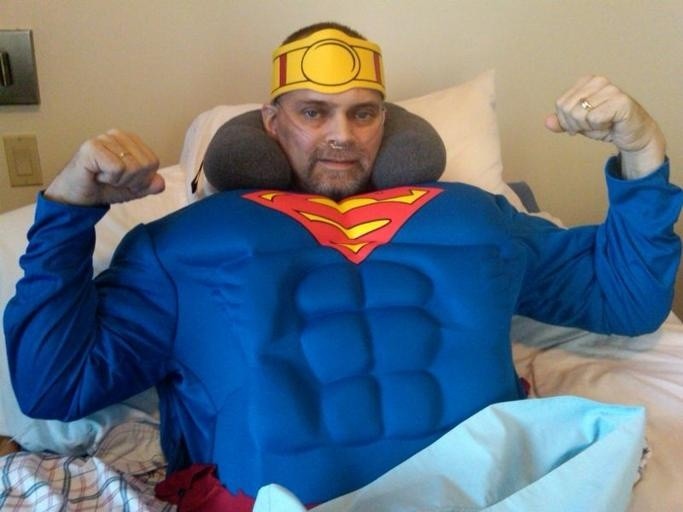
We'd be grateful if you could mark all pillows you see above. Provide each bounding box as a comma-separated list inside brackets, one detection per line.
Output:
[0, 164, 188, 460]
[179, 68, 529, 215]
[202, 103, 447, 189]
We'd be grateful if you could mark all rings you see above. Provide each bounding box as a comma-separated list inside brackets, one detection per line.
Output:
[118, 151, 130, 159]
[580, 98, 592, 112]
